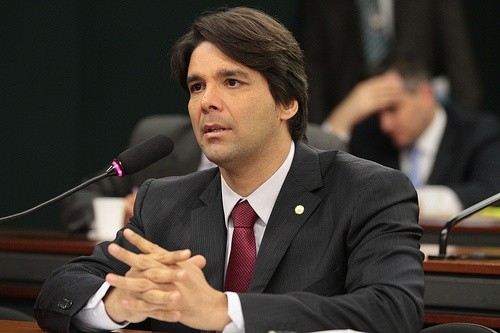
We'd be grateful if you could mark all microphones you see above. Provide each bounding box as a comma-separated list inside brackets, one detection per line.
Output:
[0, 134, 174, 224]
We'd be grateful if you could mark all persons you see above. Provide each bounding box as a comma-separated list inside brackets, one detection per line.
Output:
[62, 116, 346, 241]
[320, 54, 500, 227]
[34, 6, 425, 333]
